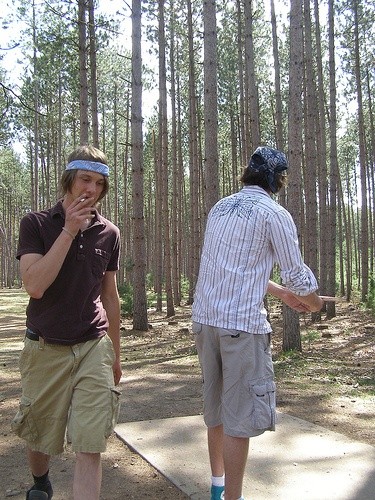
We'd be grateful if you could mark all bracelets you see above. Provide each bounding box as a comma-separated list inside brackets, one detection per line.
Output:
[62, 227, 75, 239]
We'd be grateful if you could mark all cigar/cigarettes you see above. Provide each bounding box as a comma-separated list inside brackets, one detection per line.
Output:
[80, 198, 86, 202]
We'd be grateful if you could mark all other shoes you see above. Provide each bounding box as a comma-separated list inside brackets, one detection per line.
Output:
[210, 484, 225, 500]
[26, 485, 54, 500]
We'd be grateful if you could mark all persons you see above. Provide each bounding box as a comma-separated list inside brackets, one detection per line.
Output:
[11, 145, 122, 500]
[190, 147, 323, 500]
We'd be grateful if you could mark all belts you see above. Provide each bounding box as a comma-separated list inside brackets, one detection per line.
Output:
[24, 329, 107, 346]
